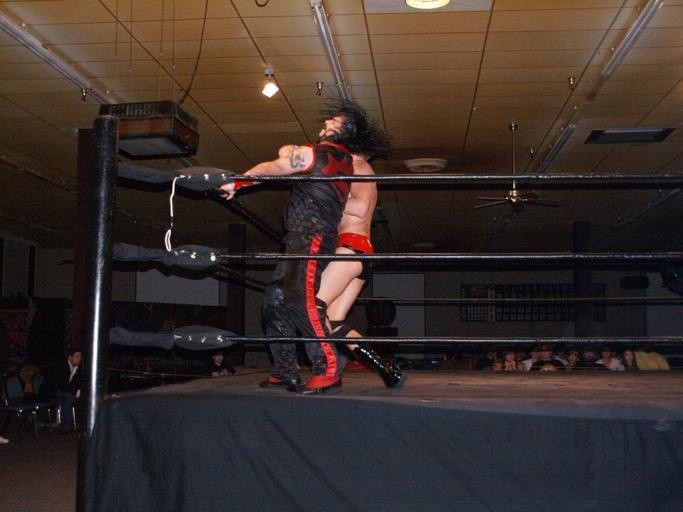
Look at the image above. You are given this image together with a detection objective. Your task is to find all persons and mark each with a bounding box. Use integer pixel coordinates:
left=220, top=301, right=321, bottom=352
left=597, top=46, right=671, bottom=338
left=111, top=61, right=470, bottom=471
left=47, top=347, right=91, bottom=432
left=314, top=131, right=409, bottom=391
left=473, top=346, right=670, bottom=372
left=207, top=350, right=234, bottom=377
left=216, top=97, right=376, bottom=395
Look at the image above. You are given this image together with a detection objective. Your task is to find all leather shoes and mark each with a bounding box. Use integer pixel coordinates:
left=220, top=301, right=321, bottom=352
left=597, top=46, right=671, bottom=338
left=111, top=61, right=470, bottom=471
left=294, top=375, right=342, bottom=396
left=256, top=371, right=302, bottom=391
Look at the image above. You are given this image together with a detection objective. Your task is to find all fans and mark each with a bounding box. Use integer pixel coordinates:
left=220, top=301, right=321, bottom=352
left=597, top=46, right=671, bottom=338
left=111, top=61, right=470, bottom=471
left=473, top=122, right=560, bottom=210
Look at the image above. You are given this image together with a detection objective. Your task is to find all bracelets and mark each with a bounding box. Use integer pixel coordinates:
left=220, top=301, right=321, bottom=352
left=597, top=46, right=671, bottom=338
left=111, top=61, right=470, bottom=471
left=232, top=174, right=254, bottom=193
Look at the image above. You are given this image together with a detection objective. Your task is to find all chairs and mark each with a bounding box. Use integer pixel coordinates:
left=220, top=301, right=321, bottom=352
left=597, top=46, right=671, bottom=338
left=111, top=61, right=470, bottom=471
left=0, top=364, right=76, bottom=442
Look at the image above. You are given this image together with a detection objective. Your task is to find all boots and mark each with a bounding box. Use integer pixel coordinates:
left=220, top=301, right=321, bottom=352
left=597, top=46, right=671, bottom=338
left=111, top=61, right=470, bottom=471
left=350, top=337, right=408, bottom=392
